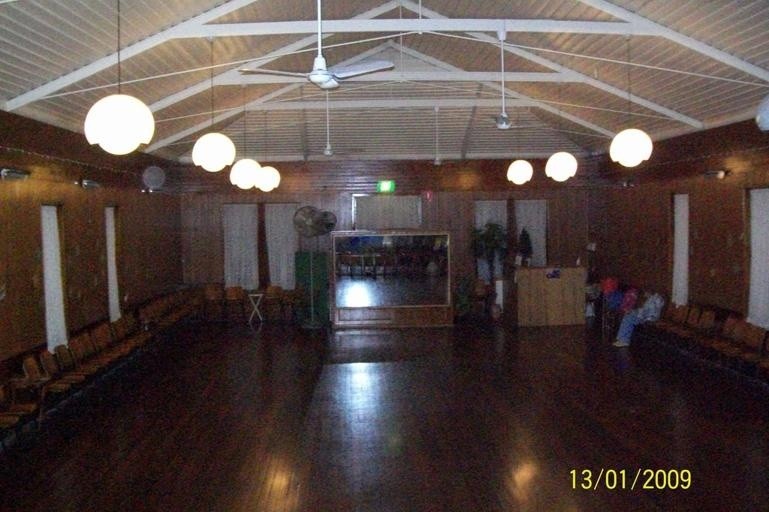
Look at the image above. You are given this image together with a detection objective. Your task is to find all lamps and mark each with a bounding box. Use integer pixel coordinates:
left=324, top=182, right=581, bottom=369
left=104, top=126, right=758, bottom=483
left=192, top=38, right=236, bottom=173
left=506, top=106, right=534, bottom=186
left=609, top=34, right=653, bottom=168
left=83, top=0, right=154, bottom=157
left=228, top=85, right=282, bottom=192
left=544, top=82, right=579, bottom=183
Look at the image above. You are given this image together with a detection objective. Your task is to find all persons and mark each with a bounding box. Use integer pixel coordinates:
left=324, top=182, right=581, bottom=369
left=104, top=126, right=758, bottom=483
left=612, top=285, right=665, bottom=348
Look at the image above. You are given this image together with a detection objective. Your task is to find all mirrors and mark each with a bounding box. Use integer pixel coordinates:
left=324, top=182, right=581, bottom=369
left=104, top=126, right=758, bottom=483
left=334, top=235, right=450, bottom=308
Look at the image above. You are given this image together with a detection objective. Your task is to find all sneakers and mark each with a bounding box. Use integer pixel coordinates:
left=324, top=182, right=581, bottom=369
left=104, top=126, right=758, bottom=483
left=611, top=340, right=630, bottom=348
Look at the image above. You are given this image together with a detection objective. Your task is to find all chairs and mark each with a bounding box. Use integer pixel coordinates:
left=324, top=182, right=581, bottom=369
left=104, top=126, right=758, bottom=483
left=612, top=282, right=769, bottom=404
left=0, top=283, right=203, bottom=452
left=203, top=282, right=306, bottom=325
left=336, top=236, right=448, bottom=281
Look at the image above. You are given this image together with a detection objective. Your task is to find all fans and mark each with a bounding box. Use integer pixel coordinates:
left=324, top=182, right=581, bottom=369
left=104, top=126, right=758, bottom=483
left=292, top=206, right=337, bottom=329
left=459, top=30, right=549, bottom=131
left=237, top=1, right=395, bottom=89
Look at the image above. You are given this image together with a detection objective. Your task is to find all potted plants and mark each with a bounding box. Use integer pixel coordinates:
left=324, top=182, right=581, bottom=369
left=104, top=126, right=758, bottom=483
left=468, top=220, right=509, bottom=325
left=450, top=274, right=488, bottom=328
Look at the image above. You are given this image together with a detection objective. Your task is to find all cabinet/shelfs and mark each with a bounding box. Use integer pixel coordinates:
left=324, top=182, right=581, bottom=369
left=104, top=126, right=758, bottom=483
left=504, top=261, right=586, bottom=328
